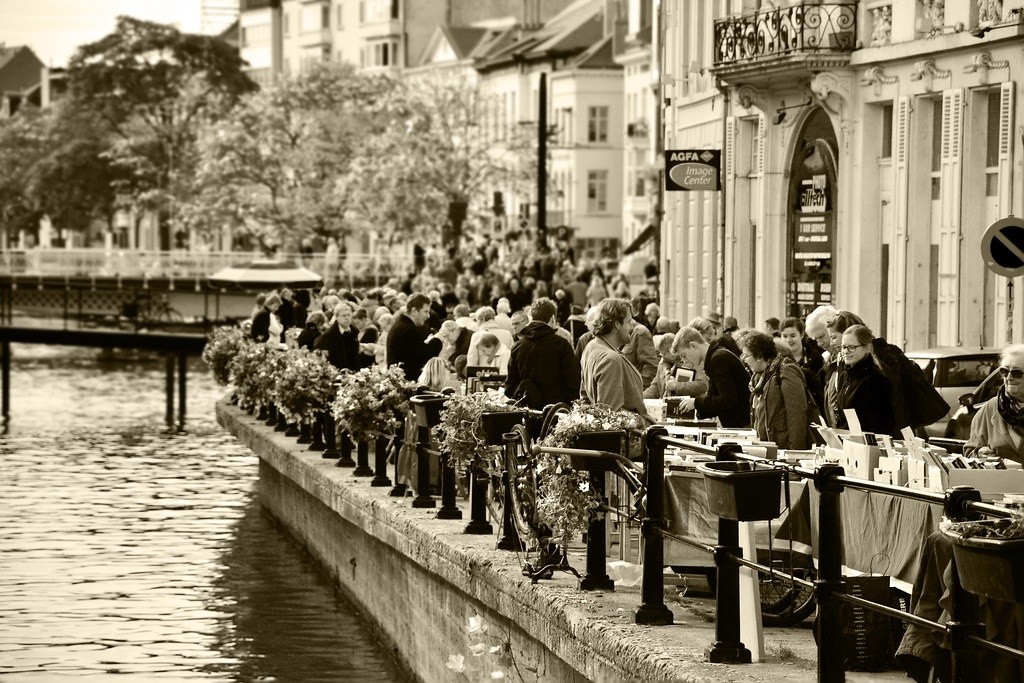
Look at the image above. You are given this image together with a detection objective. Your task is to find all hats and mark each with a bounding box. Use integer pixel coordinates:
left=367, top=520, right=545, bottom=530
left=706, top=312, right=721, bottom=325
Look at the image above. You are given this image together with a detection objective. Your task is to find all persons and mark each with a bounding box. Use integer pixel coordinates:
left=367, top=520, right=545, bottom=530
left=826, top=324, right=894, bottom=445
left=963, top=345, right=1024, bottom=466
left=825, top=308, right=932, bottom=443
left=251, top=210, right=829, bottom=430
left=804, top=305, right=848, bottom=389
left=741, top=332, right=825, bottom=451
left=579, top=297, right=667, bottom=469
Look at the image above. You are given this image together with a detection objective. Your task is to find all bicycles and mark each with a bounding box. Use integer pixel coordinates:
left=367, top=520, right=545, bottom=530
left=81, top=286, right=185, bottom=334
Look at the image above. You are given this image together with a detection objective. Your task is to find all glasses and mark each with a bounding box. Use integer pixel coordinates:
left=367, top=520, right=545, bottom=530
left=999, top=368, right=1024, bottom=379
left=838, top=344, right=863, bottom=353
left=741, top=353, right=752, bottom=359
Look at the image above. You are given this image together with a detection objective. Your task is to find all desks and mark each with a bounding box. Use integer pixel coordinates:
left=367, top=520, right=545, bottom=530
left=603, top=414, right=1024, bottom=683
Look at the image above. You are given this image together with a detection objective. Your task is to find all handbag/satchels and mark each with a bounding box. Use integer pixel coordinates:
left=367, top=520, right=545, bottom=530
left=807, top=400, right=828, bottom=445
